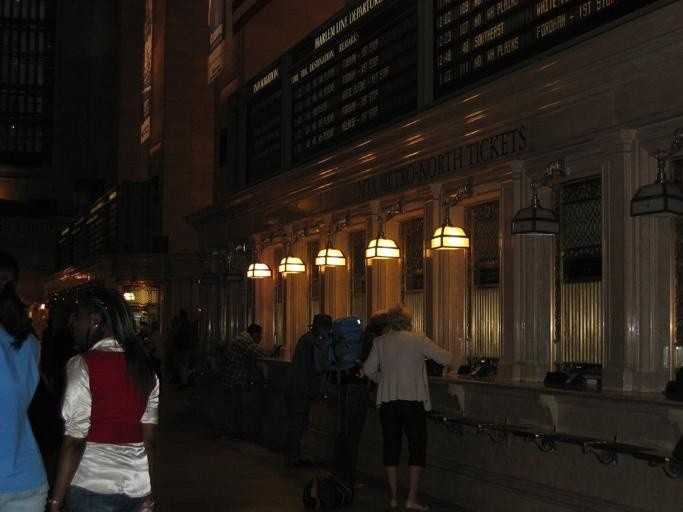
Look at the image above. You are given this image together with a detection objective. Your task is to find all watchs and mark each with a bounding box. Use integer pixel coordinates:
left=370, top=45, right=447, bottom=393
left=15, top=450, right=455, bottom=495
left=49, top=498, right=63, bottom=504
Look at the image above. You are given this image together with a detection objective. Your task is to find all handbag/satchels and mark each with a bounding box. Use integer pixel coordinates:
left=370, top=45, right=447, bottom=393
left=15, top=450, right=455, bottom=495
left=304, top=473, right=348, bottom=511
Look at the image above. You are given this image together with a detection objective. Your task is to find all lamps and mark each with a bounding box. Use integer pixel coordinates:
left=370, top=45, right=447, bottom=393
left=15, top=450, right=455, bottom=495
left=511, top=161, right=568, bottom=236
left=431, top=183, right=471, bottom=250
left=278, top=228, right=306, bottom=272
left=630, top=128, right=683, bottom=217
left=364, top=202, right=401, bottom=259
left=315, top=214, right=346, bottom=266
left=192, top=251, right=219, bottom=285
left=219, top=245, right=243, bottom=282
left=247, top=237, right=272, bottom=278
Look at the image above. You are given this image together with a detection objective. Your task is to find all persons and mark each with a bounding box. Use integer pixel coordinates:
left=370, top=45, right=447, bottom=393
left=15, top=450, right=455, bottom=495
left=325, top=310, right=388, bottom=476
left=0, top=251, right=49, bottom=511
left=222, top=322, right=282, bottom=439
left=281, top=314, right=333, bottom=467
left=42, top=317, right=73, bottom=352
left=359, top=302, right=458, bottom=512
left=50, top=287, right=160, bottom=511
left=139, top=308, right=201, bottom=390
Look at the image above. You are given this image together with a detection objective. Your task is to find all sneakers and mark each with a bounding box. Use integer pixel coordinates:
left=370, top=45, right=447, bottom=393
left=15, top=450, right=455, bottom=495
left=406, top=500, right=429, bottom=510
left=388, top=498, right=396, bottom=507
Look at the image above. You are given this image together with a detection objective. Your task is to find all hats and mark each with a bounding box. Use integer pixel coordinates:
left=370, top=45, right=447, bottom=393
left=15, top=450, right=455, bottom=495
left=307, top=314, right=332, bottom=330
